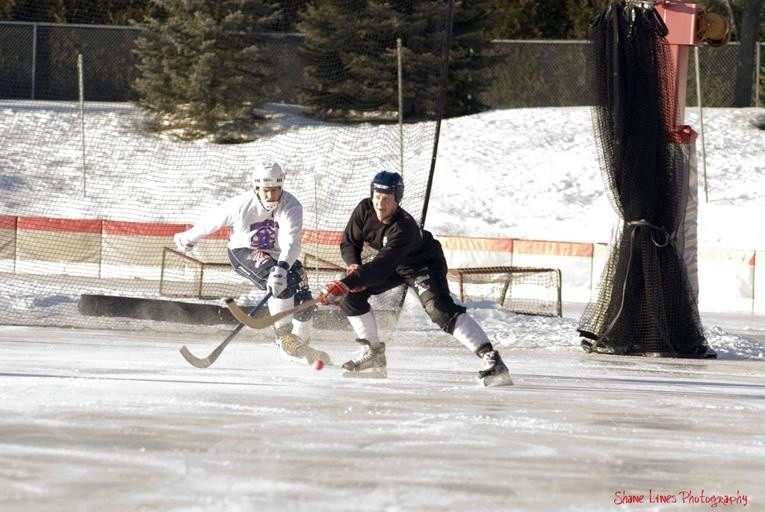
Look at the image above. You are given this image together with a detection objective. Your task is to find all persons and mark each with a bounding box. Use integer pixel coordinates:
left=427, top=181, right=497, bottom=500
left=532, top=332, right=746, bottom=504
left=173, top=160, right=511, bottom=386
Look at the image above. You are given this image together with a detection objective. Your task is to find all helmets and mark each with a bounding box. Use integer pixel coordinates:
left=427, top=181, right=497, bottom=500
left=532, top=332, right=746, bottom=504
left=371, top=171, right=405, bottom=202
left=252, top=160, right=284, bottom=192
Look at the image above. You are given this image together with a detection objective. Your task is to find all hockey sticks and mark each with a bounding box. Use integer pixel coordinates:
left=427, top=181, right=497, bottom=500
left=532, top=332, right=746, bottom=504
left=179, top=291, right=272, bottom=368
left=224, top=297, right=321, bottom=328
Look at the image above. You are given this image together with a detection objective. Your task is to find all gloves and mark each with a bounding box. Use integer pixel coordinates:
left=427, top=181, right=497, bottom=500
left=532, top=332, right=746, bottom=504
left=347, top=264, right=367, bottom=292
left=175, top=232, right=194, bottom=253
left=320, top=280, right=348, bottom=308
left=267, top=266, right=288, bottom=297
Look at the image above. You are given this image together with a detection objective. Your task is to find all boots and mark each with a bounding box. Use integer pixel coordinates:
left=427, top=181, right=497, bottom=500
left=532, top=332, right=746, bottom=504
left=478, top=350, right=509, bottom=380
left=282, top=334, right=330, bottom=364
left=342, top=338, right=386, bottom=372
